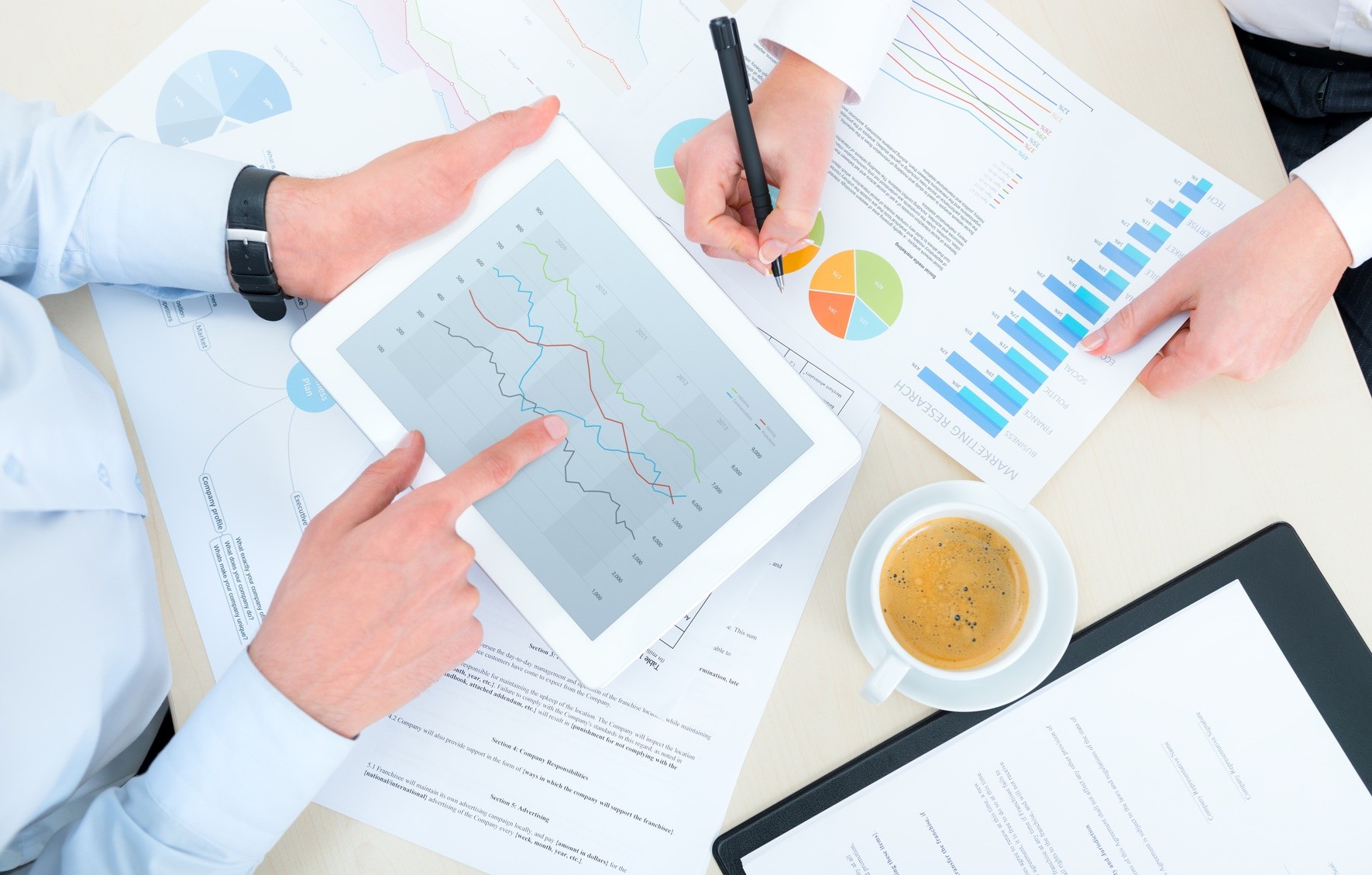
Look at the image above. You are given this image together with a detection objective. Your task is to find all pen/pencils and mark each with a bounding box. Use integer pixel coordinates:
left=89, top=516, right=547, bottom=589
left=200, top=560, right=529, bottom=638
left=709, top=15, right=786, bottom=295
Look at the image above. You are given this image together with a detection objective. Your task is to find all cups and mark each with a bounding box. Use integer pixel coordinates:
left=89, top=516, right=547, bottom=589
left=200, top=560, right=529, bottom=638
left=864, top=504, right=1050, bottom=699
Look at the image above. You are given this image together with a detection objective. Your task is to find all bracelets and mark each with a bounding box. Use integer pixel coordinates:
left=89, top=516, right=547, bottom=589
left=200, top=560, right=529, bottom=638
left=227, top=159, right=293, bottom=320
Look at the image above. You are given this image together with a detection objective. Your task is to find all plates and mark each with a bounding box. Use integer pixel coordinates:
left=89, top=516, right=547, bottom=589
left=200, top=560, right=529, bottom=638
left=845, top=479, right=1080, bottom=712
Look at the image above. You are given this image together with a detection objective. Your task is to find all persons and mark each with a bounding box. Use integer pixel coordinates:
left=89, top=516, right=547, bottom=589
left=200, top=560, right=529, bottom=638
left=0, top=84, right=576, bottom=875
left=674, top=0, right=1372, bottom=406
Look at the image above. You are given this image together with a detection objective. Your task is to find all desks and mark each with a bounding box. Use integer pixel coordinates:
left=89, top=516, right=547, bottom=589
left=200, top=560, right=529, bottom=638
left=0, top=6, right=1372, bottom=875
